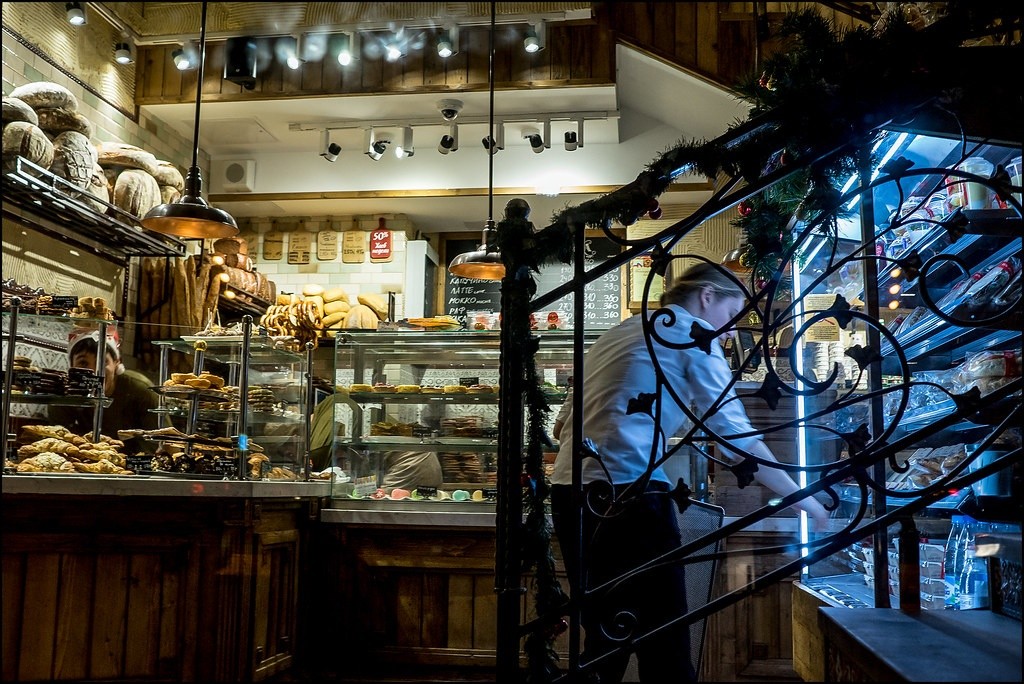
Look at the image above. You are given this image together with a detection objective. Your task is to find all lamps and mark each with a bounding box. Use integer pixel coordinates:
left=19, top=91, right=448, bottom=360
left=438, top=121, right=459, bottom=155
left=338, top=31, right=361, bottom=66
left=448, top=1, right=507, bottom=279
left=526, top=119, right=551, bottom=153
left=320, top=130, right=341, bottom=162
left=287, top=35, right=308, bottom=69
left=364, top=130, right=391, bottom=161
left=65, top=2, right=88, bottom=25
left=523, top=22, right=546, bottom=53
left=396, top=128, right=415, bottom=159
left=172, top=49, right=189, bottom=70
left=565, top=120, right=584, bottom=151
left=437, top=27, right=460, bottom=57
left=141, top=1, right=240, bottom=239
left=482, top=124, right=505, bottom=155
left=115, top=41, right=135, bottom=64
left=385, top=32, right=410, bottom=61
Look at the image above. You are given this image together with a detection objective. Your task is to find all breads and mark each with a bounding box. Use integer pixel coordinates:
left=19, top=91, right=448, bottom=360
left=261, top=282, right=388, bottom=338
left=348, top=382, right=499, bottom=395
left=948, top=348, right=1024, bottom=398
left=2, top=80, right=337, bottom=479
left=906, top=452, right=969, bottom=487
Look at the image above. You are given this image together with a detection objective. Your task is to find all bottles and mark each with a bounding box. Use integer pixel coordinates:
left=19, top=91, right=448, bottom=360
left=943, top=515, right=1021, bottom=610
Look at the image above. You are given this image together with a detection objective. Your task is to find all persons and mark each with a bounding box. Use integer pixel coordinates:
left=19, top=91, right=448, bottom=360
left=263, top=395, right=442, bottom=493
left=49, top=311, right=175, bottom=440
left=550, top=262, right=828, bottom=684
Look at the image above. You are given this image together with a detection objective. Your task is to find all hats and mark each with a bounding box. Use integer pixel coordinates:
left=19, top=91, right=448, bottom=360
left=69, top=323, right=125, bottom=375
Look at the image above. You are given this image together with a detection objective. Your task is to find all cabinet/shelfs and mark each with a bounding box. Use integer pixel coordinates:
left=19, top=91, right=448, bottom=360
left=335, top=330, right=708, bottom=507
left=149, top=337, right=308, bottom=478
left=788, top=129, right=1024, bottom=683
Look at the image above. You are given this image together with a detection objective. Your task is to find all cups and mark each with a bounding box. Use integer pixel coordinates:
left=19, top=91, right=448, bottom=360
left=965, top=443, right=1012, bottom=496
left=1006, top=156, right=1022, bottom=207
left=942, top=157, right=994, bottom=210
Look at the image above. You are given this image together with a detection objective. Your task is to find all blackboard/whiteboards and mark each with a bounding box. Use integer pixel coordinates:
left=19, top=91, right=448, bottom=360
left=437, top=228, right=631, bottom=330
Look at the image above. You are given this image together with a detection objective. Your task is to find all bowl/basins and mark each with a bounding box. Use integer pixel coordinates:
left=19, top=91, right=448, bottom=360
left=879, top=189, right=947, bottom=265
left=815, top=328, right=868, bottom=390
left=827, top=261, right=864, bottom=302
left=669, top=438, right=683, bottom=445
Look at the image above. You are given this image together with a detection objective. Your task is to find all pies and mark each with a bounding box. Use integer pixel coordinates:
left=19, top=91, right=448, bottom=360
left=437, top=415, right=497, bottom=483
left=407, top=317, right=459, bottom=327
